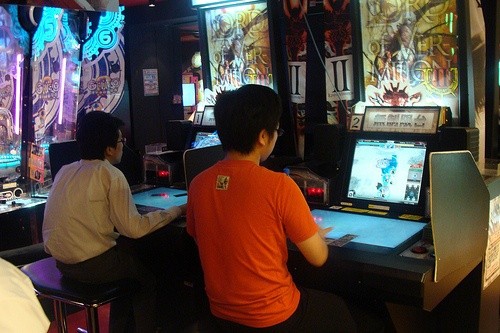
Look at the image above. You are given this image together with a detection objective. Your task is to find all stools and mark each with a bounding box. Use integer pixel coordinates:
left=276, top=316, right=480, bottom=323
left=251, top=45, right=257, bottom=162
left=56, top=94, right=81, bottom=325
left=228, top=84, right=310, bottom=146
left=23, top=257, right=125, bottom=333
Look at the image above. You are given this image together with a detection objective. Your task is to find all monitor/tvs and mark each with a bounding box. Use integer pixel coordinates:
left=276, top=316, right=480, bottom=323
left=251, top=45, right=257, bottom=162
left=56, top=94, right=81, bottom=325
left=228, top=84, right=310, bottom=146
left=343, top=137, right=432, bottom=211
left=187, top=130, right=222, bottom=150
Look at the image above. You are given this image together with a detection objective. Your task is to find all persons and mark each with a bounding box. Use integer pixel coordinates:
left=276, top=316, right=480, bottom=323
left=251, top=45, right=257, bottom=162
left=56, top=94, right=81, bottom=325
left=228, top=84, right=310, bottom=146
left=43, top=110, right=199, bottom=333
left=187, top=84, right=387, bottom=333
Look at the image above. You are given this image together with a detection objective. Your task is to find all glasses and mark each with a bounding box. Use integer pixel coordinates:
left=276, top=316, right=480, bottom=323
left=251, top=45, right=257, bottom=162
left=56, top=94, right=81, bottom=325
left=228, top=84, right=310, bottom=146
left=116, top=138, right=126, bottom=145
left=274, top=128, right=284, bottom=136
